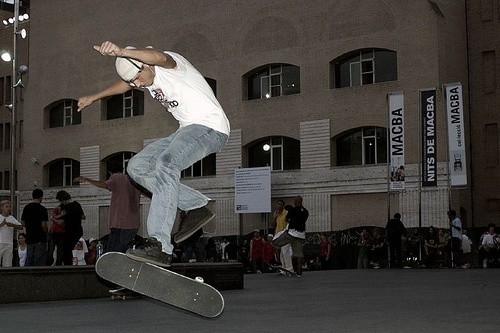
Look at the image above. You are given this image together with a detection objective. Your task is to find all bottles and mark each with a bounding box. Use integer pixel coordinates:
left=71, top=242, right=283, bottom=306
left=96, top=240, right=103, bottom=260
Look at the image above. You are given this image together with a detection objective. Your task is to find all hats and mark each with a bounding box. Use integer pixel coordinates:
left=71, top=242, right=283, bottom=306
left=115, top=46, right=143, bottom=81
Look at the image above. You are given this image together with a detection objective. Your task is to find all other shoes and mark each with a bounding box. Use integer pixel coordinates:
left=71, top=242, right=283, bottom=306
left=483, top=259, right=487, bottom=267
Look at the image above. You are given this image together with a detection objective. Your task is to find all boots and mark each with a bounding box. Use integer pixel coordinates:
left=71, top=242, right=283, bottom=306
left=292, top=255, right=304, bottom=277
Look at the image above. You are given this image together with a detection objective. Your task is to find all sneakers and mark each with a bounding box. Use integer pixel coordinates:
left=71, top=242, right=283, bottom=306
left=174, top=206, right=215, bottom=243
left=126, top=237, right=171, bottom=267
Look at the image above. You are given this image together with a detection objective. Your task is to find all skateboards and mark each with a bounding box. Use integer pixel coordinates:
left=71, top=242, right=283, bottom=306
left=95, top=252, right=225, bottom=318
left=268, top=264, right=304, bottom=278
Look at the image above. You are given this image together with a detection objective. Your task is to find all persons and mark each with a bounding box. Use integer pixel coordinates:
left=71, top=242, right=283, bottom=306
left=369, top=228, right=384, bottom=269
left=273, top=199, right=294, bottom=277
left=447, top=209, right=471, bottom=268
left=400, top=226, right=473, bottom=268
left=0, top=200, right=23, bottom=267
left=218, top=229, right=331, bottom=274
left=272, top=195, right=309, bottom=277
left=479, top=223, right=500, bottom=269
left=21, top=189, right=50, bottom=266
left=71, top=237, right=88, bottom=266
left=49, top=203, right=67, bottom=267
left=1, top=238, right=20, bottom=267
left=51, top=190, right=86, bottom=266
left=18, top=233, right=28, bottom=267
left=358, top=228, right=371, bottom=269
left=77, top=40, right=231, bottom=268
left=84, top=238, right=97, bottom=265
left=73, top=159, right=152, bottom=292
left=385, top=212, right=409, bottom=269
